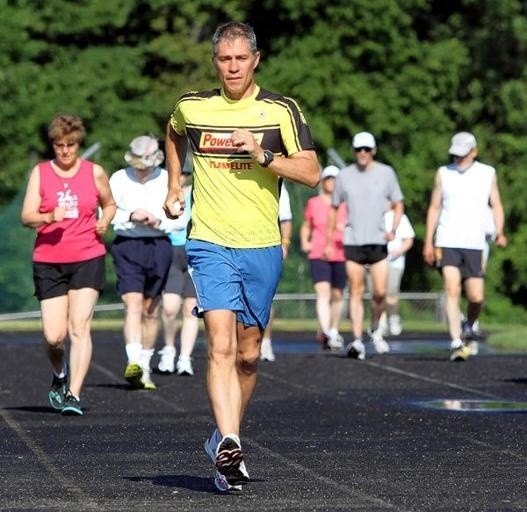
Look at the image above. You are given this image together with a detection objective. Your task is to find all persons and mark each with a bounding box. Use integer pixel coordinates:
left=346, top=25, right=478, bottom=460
left=421, top=130, right=509, bottom=362
left=364, top=195, right=417, bottom=340
left=298, top=164, right=349, bottom=352
left=322, top=131, right=405, bottom=362
left=155, top=158, right=205, bottom=377
left=96, top=134, right=183, bottom=392
left=161, top=18, right=322, bottom=492
left=255, top=178, right=293, bottom=363
left=20, top=110, right=119, bottom=416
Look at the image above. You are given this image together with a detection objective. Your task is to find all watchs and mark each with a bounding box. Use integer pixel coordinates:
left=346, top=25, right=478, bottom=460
left=258, top=149, right=275, bottom=170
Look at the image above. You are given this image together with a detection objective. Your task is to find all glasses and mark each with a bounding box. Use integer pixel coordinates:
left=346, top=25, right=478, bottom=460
left=354, top=147, right=373, bottom=152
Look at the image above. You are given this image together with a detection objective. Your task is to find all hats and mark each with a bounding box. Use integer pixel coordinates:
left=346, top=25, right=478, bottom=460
left=321, top=165, right=340, bottom=180
left=352, top=132, right=376, bottom=149
left=124, top=136, right=164, bottom=170
left=448, top=131, right=476, bottom=157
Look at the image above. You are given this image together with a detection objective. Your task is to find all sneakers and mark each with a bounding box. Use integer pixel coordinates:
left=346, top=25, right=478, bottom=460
left=158, top=346, right=176, bottom=373
left=204, top=429, right=243, bottom=492
left=140, top=378, right=156, bottom=390
left=124, top=363, right=145, bottom=388
left=49, top=360, right=68, bottom=410
left=260, top=338, right=275, bottom=361
left=61, top=395, right=83, bottom=415
left=176, top=356, right=194, bottom=376
left=215, top=436, right=251, bottom=485
left=317, top=313, right=478, bottom=362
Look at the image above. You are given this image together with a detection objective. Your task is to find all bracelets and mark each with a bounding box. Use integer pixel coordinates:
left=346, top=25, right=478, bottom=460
left=281, top=238, right=291, bottom=247
left=391, top=229, right=397, bottom=234
